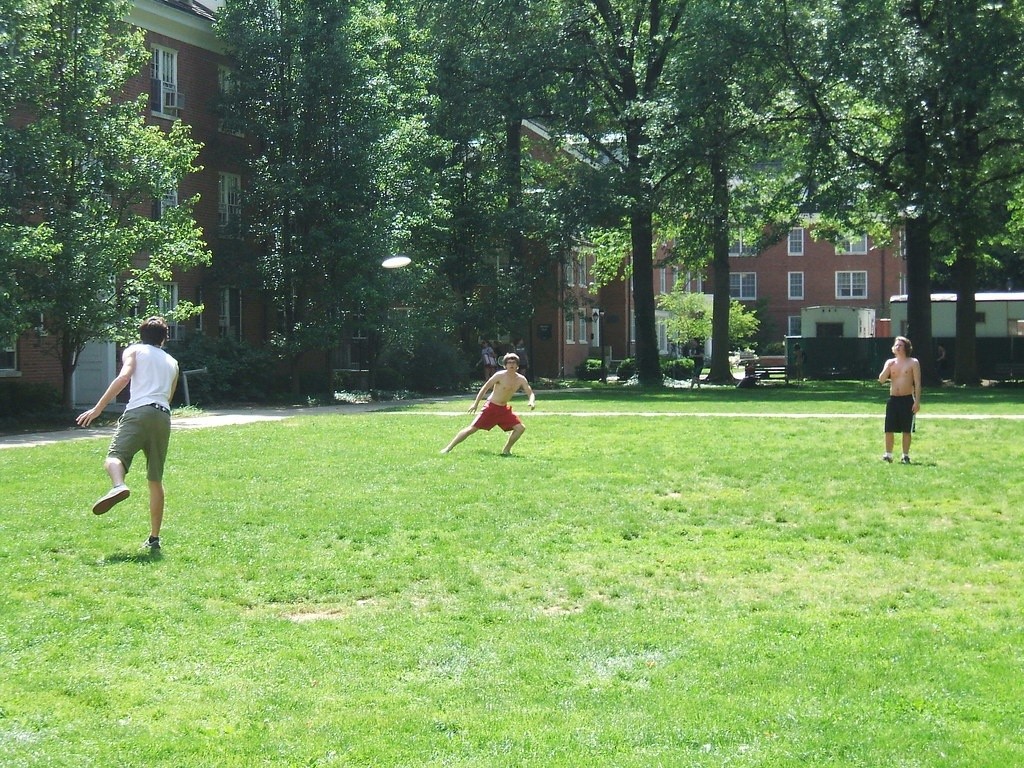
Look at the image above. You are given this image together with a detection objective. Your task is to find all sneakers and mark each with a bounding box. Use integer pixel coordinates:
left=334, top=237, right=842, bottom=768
left=93, top=485, right=130, bottom=516
left=143, top=538, right=162, bottom=549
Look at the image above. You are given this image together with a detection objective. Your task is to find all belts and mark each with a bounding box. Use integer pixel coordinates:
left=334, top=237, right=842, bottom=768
left=151, top=403, right=171, bottom=416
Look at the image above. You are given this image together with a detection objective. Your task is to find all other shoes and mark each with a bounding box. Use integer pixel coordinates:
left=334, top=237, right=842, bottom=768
left=882, top=456, right=893, bottom=463
left=900, top=456, right=911, bottom=465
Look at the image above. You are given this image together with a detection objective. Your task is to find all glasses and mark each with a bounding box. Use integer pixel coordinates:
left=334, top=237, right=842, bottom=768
left=506, top=361, right=518, bottom=364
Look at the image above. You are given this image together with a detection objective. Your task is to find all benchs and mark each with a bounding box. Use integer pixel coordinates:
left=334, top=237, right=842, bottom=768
left=744, top=365, right=788, bottom=386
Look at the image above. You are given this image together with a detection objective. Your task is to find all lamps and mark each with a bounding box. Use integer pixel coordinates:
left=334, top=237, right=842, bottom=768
left=588, top=311, right=598, bottom=323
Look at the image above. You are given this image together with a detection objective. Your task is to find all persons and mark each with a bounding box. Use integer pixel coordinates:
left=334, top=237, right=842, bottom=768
left=878, top=336, right=922, bottom=464
left=793, top=343, right=807, bottom=386
left=688, top=338, right=704, bottom=392
left=440, top=353, right=535, bottom=456
left=475, top=339, right=497, bottom=392
left=511, top=337, right=529, bottom=390
left=76, top=316, right=180, bottom=550
